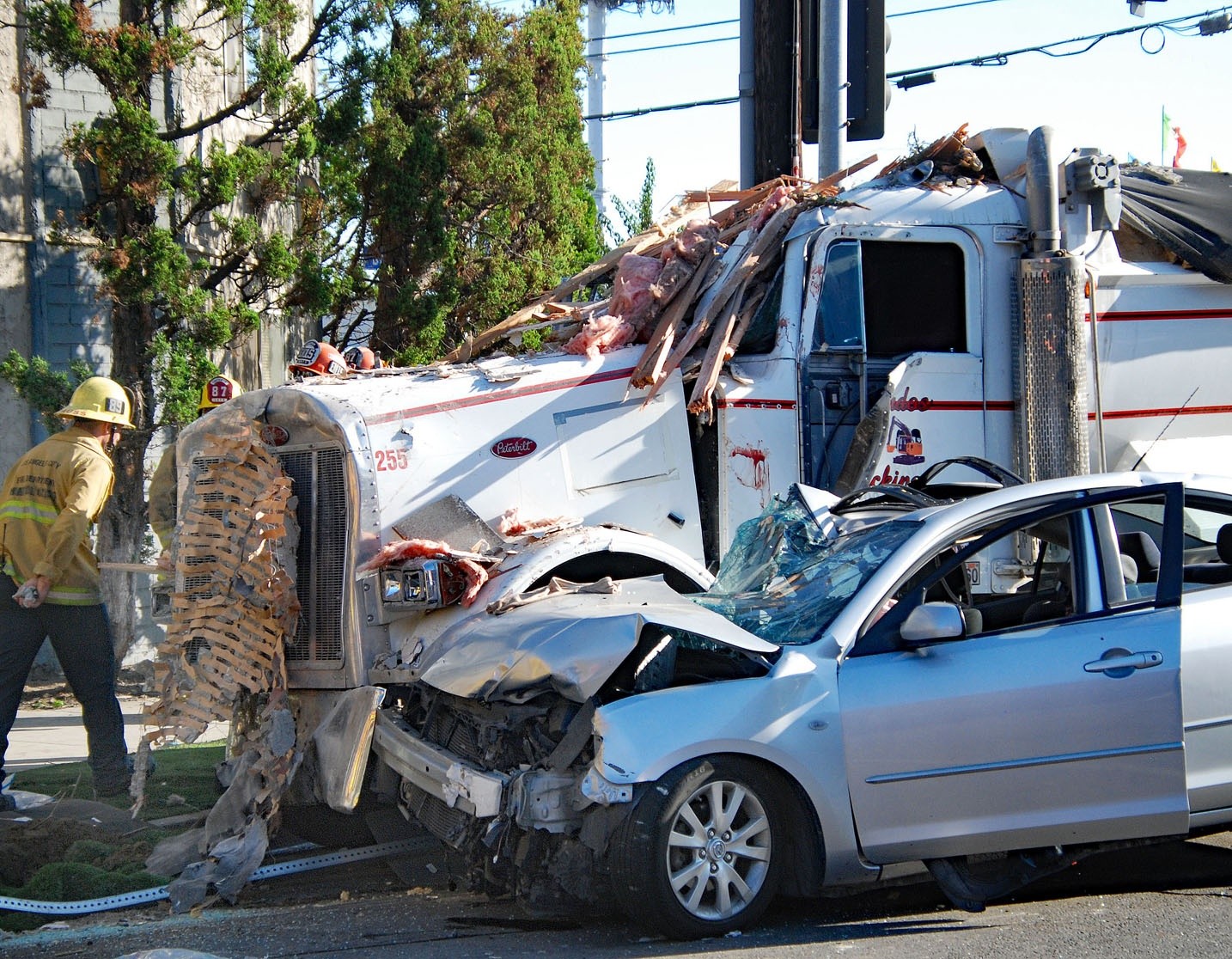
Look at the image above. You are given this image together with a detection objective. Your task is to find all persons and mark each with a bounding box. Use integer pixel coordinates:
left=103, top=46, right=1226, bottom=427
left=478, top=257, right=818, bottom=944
left=149, top=375, right=242, bottom=583
left=288, top=339, right=385, bottom=383
left=0, top=377, right=158, bottom=812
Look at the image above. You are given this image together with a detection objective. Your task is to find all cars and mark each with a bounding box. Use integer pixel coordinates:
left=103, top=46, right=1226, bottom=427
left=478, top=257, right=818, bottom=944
left=372, top=472, right=1232, bottom=941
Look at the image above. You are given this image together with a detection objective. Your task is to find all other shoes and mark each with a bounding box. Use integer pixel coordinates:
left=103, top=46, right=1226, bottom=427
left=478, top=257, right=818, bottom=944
left=95, top=753, right=156, bottom=797
left=0, top=790, right=16, bottom=811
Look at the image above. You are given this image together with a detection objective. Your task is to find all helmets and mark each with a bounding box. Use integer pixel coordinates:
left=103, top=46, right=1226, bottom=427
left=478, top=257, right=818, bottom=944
left=289, top=339, right=347, bottom=376
left=197, top=374, right=244, bottom=411
left=340, top=345, right=375, bottom=374
left=53, top=376, right=136, bottom=429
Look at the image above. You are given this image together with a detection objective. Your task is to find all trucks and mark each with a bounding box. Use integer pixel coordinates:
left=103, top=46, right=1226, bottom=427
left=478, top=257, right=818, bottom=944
left=172, top=125, right=1230, bottom=871
